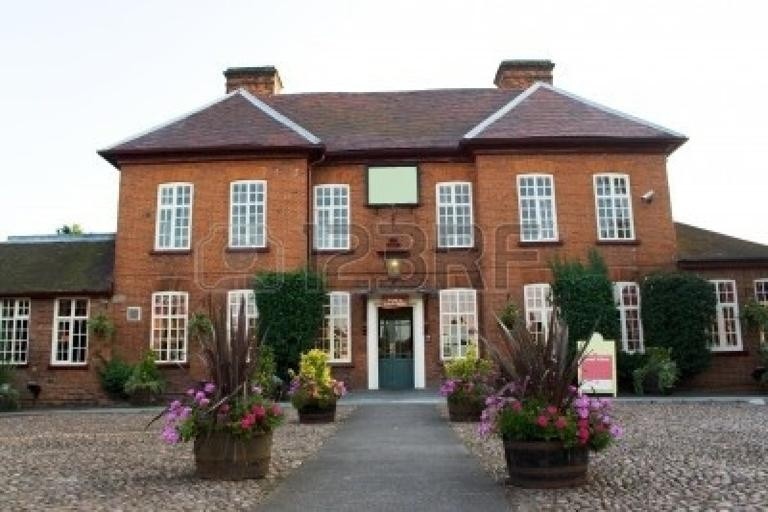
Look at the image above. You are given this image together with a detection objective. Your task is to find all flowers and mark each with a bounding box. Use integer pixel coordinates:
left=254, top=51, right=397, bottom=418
left=440, top=343, right=499, bottom=410
left=474, top=304, right=624, bottom=453
left=145, top=294, right=284, bottom=446
left=287, top=349, right=348, bottom=408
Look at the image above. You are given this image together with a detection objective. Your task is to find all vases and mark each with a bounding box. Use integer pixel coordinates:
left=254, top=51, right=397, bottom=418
left=448, top=399, right=483, bottom=423
left=503, top=439, right=589, bottom=490
left=298, top=405, right=336, bottom=424
left=194, top=434, right=271, bottom=481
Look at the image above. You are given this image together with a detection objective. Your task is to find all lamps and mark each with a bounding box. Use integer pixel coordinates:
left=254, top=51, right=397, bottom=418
left=377, top=250, right=410, bottom=284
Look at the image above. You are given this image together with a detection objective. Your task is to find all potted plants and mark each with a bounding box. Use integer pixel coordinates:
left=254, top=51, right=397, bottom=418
left=0, top=363, right=21, bottom=412
left=632, top=346, right=679, bottom=395
left=124, top=350, right=165, bottom=406
